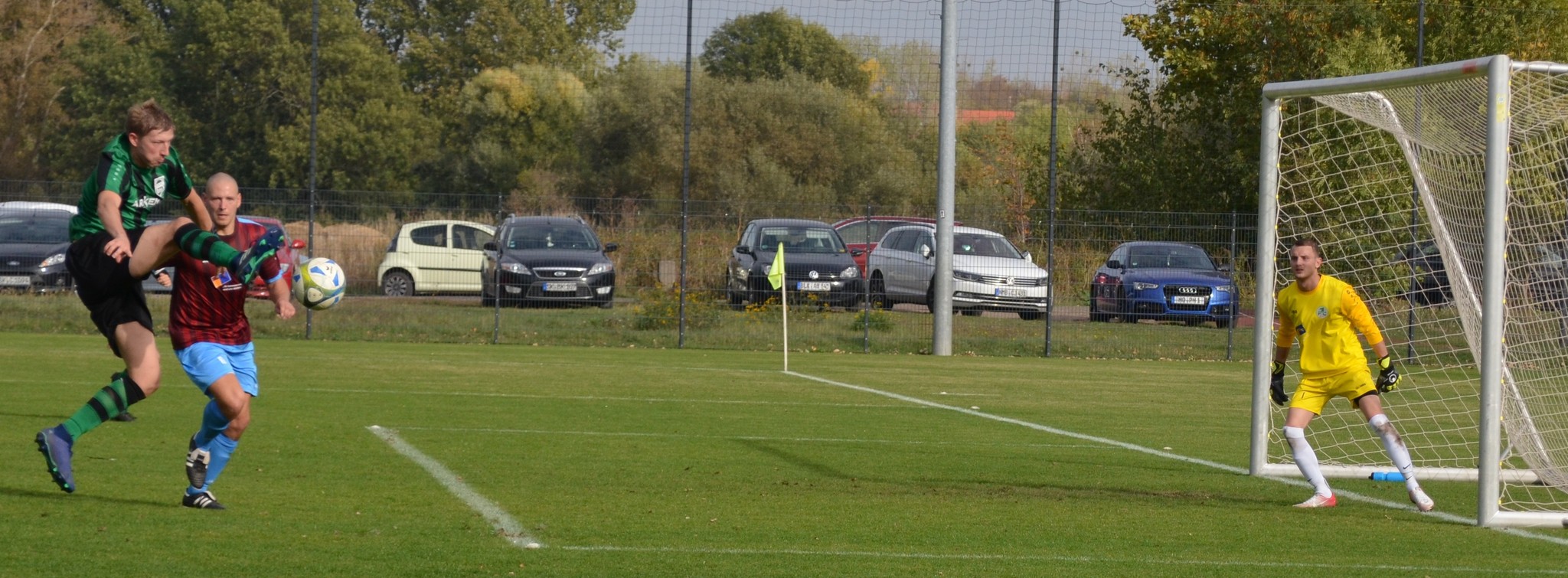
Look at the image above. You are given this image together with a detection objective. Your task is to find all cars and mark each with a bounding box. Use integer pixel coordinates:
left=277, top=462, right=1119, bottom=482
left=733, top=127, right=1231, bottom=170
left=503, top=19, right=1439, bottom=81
left=481, top=213, right=615, bottom=308
left=1089, top=241, right=1249, bottom=327
left=725, top=219, right=864, bottom=311
left=866, top=223, right=1055, bottom=320
left=377, top=220, right=499, bottom=298
left=236, top=214, right=307, bottom=298
left=0, top=208, right=77, bottom=295
left=833, top=218, right=964, bottom=281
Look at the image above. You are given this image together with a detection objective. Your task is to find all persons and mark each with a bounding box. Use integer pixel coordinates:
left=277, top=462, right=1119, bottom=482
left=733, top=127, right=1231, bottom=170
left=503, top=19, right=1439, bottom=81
left=149, top=171, right=296, bottom=510
left=35, top=97, right=285, bottom=494
left=1270, top=239, right=1434, bottom=513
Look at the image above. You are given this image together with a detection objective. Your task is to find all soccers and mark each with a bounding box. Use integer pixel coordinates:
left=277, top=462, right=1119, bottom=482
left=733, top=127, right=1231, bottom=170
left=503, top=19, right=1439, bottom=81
left=293, top=258, right=346, bottom=310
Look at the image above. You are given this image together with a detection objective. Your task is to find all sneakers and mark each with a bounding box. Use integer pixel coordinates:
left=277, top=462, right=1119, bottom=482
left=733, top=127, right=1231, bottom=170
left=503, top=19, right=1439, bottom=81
left=235, top=227, right=286, bottom=286
left=1408, top=487, right=1434, bottom=511
left=1292, top=492, right=1336, bottom=508
left=182, top=489, right=225, bottom=509
left=35, top=427, right=74, bottom=493
left=186, top=430, right=211, bottom=489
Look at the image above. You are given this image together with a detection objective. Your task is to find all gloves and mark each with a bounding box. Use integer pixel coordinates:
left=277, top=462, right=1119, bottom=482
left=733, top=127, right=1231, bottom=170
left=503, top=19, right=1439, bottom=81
left=1375, top=356, right=1402, bottom=394
left=1270, top=361, right=1288, bottom=406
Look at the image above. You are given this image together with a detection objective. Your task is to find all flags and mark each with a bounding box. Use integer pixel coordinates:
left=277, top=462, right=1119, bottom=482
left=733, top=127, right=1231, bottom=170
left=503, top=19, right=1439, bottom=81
left=767, top=242, right=785, bottom=291
